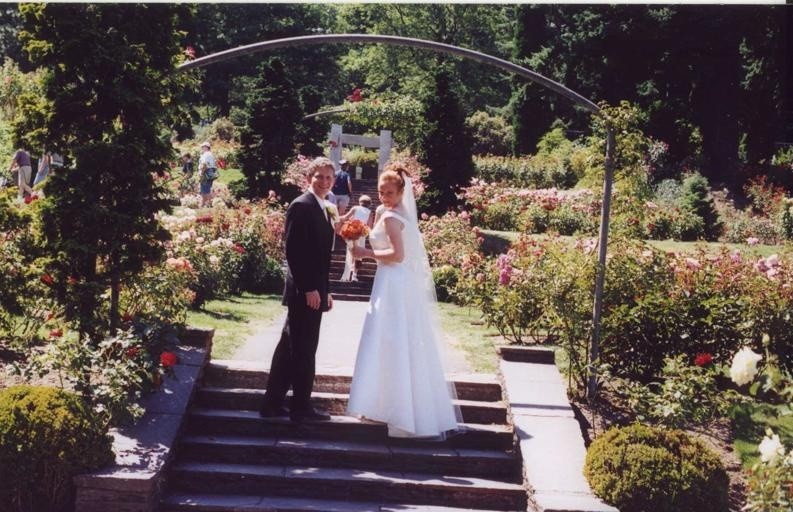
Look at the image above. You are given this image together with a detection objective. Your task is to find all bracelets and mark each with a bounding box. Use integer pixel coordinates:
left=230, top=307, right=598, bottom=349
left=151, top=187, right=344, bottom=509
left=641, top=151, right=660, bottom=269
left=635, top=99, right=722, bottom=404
left=369, top=249, right=374, bottom=257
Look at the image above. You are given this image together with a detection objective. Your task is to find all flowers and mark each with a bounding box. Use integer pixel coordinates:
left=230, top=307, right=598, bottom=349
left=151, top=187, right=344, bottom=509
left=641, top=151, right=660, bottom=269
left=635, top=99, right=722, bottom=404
left=332, top=217, right=371, bottom=280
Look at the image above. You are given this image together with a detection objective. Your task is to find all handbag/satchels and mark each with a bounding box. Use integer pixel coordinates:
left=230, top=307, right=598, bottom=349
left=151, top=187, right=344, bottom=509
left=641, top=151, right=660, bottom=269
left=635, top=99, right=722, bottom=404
left=205, top=168, right=219, bottom=180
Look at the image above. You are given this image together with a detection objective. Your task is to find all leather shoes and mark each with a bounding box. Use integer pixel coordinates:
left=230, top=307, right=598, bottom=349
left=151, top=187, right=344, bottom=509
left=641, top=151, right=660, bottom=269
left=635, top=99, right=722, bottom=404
left=260, top=408, right=290, bottom=417
left=290, top=410, right=330, bottom=421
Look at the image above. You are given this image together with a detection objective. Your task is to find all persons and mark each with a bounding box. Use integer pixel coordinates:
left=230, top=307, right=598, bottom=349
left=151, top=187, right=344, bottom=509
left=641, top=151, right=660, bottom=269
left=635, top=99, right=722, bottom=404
left=324, top=159, right=373, bottom=283
left=260, top=156, right=336, bottom=424
left=344, top=164, right=468, bottom=443
left=181, top=151, right=193, bottom=177
left=197, top=142, right=217, bottom=208
left=7, top=147, right=63, bottom=204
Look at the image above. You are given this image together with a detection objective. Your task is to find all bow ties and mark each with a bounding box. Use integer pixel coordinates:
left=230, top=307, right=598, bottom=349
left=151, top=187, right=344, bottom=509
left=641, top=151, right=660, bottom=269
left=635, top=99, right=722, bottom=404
left=318, top=199, right=336, bottom=210
